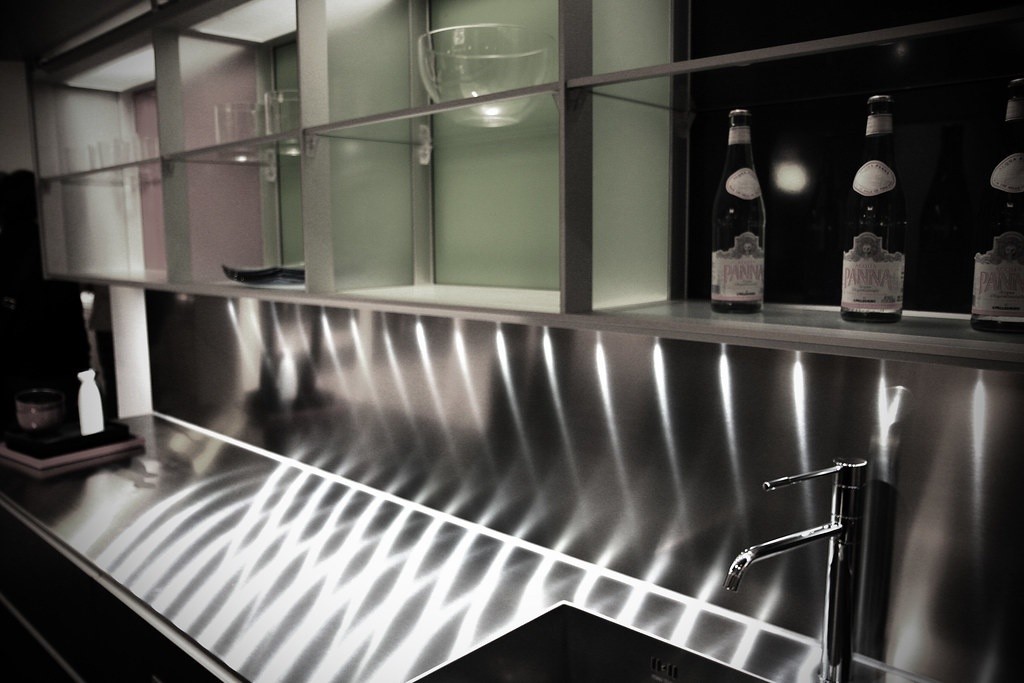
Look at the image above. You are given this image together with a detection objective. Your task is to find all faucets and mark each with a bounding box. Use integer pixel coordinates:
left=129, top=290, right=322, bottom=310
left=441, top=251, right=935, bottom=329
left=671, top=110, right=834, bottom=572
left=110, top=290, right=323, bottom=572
left=724, top=455, right=870, bottom=683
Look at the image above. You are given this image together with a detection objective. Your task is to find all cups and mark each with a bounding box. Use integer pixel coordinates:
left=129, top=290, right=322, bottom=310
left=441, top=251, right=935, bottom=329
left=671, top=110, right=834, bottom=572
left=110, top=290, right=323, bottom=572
left=266, top=92, right=301, bottom=154
left=44, top=134, right=157, bottom=175
left=214, top=102, right=258, bottom=160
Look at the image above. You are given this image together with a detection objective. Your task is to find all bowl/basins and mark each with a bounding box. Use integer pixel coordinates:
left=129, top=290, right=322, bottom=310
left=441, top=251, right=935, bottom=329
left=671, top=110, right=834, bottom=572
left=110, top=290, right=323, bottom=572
left=16, top=389, right=67, bottom=430
left=417, top=26, right=555, bottom=128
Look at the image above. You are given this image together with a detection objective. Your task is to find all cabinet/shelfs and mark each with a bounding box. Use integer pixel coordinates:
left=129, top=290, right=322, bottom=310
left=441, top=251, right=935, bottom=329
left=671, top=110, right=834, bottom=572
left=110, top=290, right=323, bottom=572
left=0, top=0, right=1023, bottom=367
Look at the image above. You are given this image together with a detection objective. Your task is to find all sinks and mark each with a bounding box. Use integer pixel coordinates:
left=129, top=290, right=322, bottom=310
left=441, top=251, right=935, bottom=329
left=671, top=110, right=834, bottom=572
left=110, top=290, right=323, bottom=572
left=402, top=600, right=774, bottom=683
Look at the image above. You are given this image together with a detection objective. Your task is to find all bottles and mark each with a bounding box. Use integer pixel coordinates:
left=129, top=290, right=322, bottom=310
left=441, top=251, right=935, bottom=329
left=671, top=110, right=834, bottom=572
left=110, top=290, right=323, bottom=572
left=970, top=76, right=1024, bottom=333
left=711, top=109, right=765, bottom=314
left=78, top=372, right=104, bottom=435
left=839, top=95, right=904, bottom=322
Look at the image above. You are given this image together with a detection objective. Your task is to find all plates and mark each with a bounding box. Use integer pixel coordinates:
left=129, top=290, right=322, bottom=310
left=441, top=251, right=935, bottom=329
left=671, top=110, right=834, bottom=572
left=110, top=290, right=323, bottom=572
left=223, top=265, right=305, bottom=285
